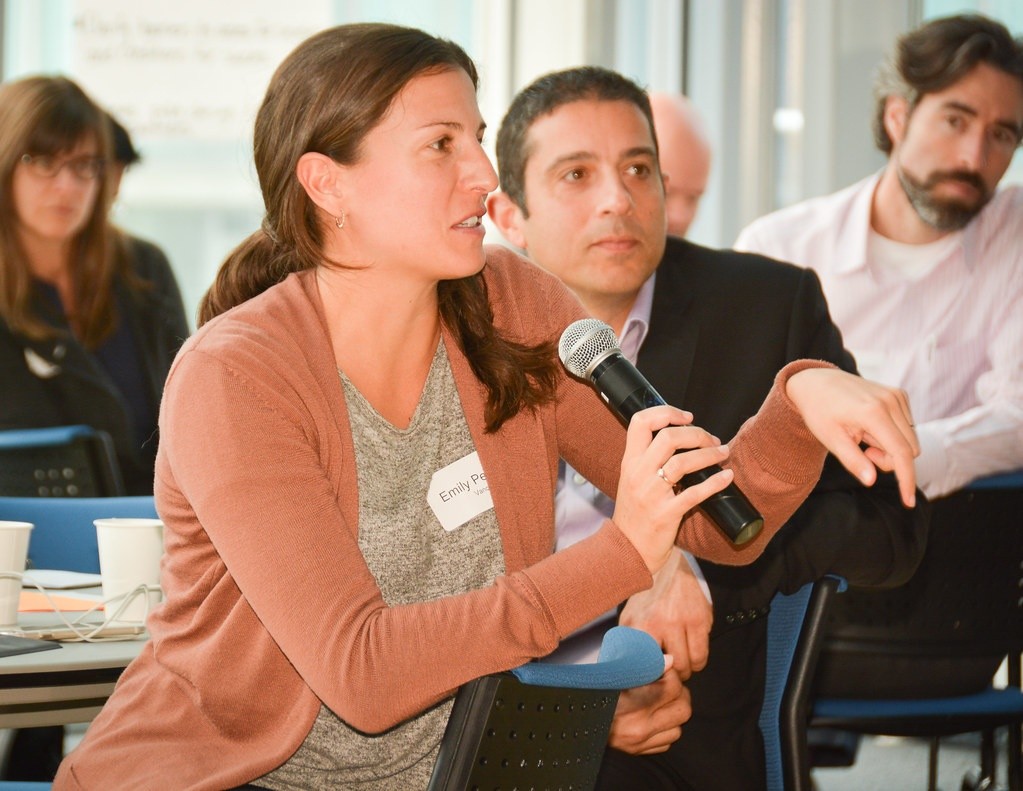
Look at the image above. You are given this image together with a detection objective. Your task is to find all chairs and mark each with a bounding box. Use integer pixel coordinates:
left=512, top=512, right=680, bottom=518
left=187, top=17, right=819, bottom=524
left=1, top=423, right=1023, bottom=789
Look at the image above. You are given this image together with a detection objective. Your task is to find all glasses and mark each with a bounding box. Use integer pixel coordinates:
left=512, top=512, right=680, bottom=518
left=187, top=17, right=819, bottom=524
left=19, top=151, right=103, bottom=180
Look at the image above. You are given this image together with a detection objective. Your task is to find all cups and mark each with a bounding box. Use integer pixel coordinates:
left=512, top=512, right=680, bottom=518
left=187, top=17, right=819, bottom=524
left=94, top=516, right=168, bottom=628
left=0, top=519, right=35, bottom=627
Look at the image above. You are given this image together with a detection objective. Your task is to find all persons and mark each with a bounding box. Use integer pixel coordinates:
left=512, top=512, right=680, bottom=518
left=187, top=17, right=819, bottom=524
left=0, top=74, right=194, bottom=497
left=50, top=12, right=1022, bottom=791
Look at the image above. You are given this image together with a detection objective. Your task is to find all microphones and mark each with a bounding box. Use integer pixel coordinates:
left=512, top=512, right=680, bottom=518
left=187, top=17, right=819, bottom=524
left=557, top=318, right=766, bottom=552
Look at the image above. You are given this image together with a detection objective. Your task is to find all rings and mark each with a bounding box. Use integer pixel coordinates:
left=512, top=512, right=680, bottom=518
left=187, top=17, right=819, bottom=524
left=657, top=467, right=677, bottom=487
